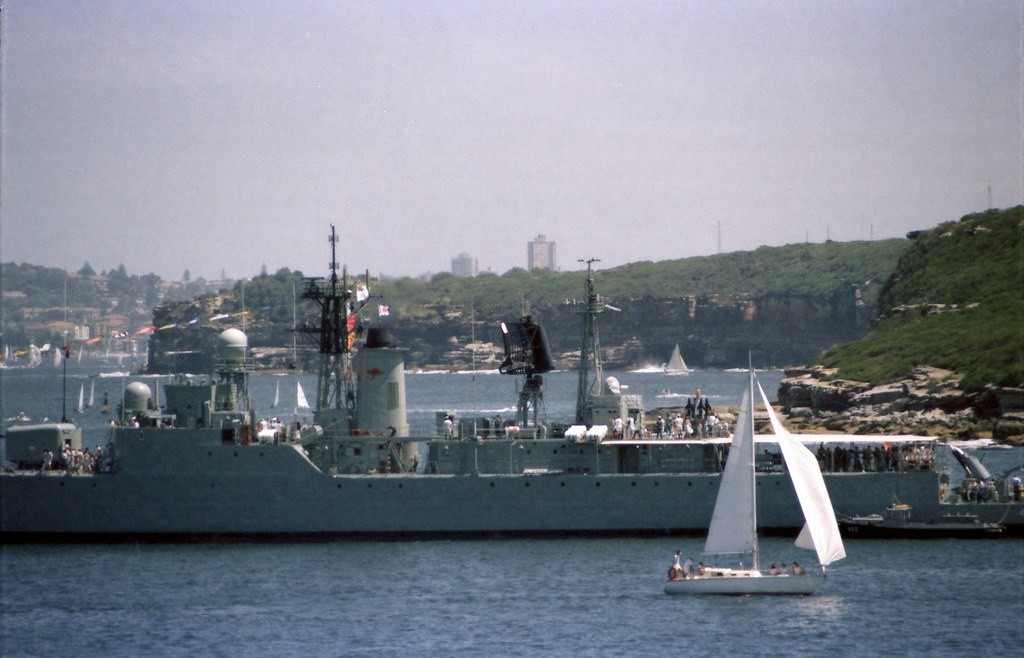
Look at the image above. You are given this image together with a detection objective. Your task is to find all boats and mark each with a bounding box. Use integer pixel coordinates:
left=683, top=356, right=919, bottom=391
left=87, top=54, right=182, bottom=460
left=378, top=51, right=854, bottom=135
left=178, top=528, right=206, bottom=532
left=0, top=224, right=1024, bottom=542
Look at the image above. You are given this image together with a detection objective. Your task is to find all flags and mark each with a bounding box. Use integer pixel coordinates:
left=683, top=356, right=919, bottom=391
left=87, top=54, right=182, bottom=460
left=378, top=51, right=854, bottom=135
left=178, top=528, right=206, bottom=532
left=355, top=284, right=369, bottom=301
left=378, top=304, right=390, bottom=317
left=346, top=302, right=356, bottom=350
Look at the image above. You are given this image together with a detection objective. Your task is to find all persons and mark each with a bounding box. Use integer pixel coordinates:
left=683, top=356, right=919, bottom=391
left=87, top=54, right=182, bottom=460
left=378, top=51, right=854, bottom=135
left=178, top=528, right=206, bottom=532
left=964, top=474, right=1024, bottom=503
left=612, top=412, right=723, bottom=441
left=442, top=415, right=453, bottom=441
left=40, top=444, right=106, bottom=474
left=494, top=414, right=505, bottom=440
left=110, top=416, right=173, bottom=429
left=816, top=442, right=936, bottom=473
left=670, top=550, right=807, bottom=577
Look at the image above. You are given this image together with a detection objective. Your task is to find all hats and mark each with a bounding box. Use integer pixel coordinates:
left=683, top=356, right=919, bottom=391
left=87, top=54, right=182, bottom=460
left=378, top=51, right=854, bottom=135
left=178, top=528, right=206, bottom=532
left=677, top=414, right=681, bottom=416
left=658, top=416, right=662, bottom=418
left=445, top=416, right=449, bottom=418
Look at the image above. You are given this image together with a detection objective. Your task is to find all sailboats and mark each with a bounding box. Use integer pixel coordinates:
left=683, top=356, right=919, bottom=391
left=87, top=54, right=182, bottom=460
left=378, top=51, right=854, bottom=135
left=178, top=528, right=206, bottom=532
left=662, top=349, right=848, bottom=596
left=661, top=344, right=695, bottom=377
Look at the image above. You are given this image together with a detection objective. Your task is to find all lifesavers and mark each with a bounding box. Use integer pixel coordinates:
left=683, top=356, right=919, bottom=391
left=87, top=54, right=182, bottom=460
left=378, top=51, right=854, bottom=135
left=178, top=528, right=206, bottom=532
left=667, top=566, right=678, bottom=580
